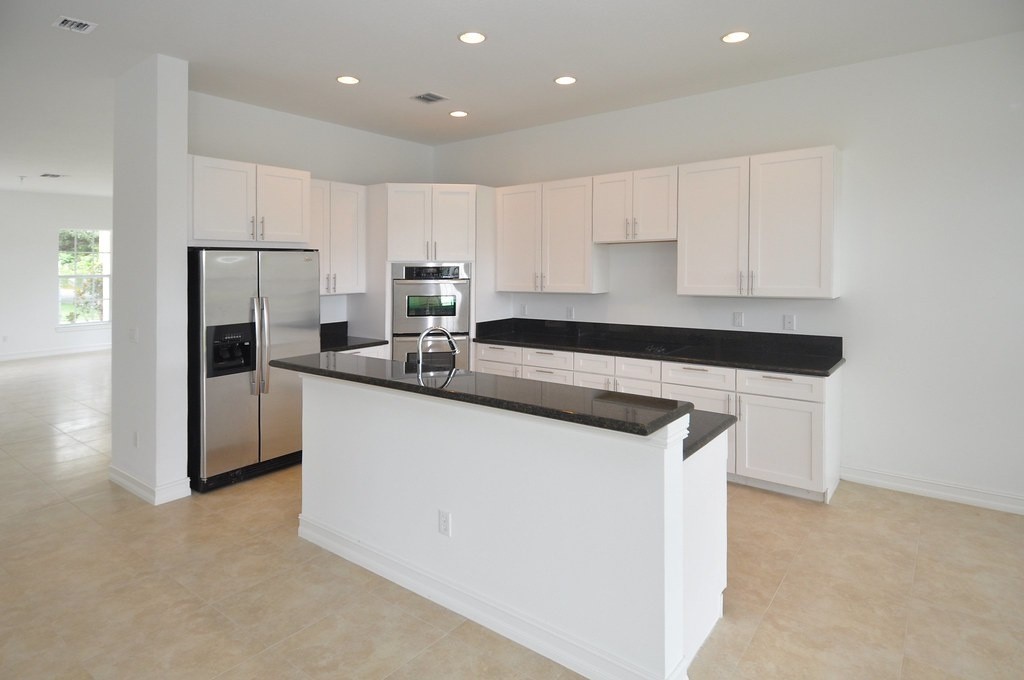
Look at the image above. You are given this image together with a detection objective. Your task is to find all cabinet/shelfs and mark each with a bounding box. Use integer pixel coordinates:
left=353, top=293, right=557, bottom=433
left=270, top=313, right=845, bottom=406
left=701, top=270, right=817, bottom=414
left=475, top=342, right=846, bottom=504
left=334, top=342, right=391, bottom=358
left=186, top=143, right=851, bottom=298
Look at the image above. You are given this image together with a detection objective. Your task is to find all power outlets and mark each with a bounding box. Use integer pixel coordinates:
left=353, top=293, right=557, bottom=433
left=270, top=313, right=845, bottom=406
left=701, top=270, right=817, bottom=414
left=783, top=313, right=795, bottom=331
left=732, top=310, right=744, bottom=328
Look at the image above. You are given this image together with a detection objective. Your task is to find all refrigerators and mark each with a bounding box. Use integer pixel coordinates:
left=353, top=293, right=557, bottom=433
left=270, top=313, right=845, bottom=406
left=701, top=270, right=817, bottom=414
left=187, top=249, right=321, bottom=494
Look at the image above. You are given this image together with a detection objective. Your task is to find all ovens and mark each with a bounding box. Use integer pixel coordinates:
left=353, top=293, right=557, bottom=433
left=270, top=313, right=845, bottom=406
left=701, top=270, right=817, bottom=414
left=391, top=261, right=470, bottom=372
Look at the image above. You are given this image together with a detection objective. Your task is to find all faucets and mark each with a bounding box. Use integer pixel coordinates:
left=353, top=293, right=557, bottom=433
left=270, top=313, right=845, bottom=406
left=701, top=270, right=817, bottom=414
left=417, top=364, right=459, bottom=391
left=416, top=326, right=460, bottom=364
